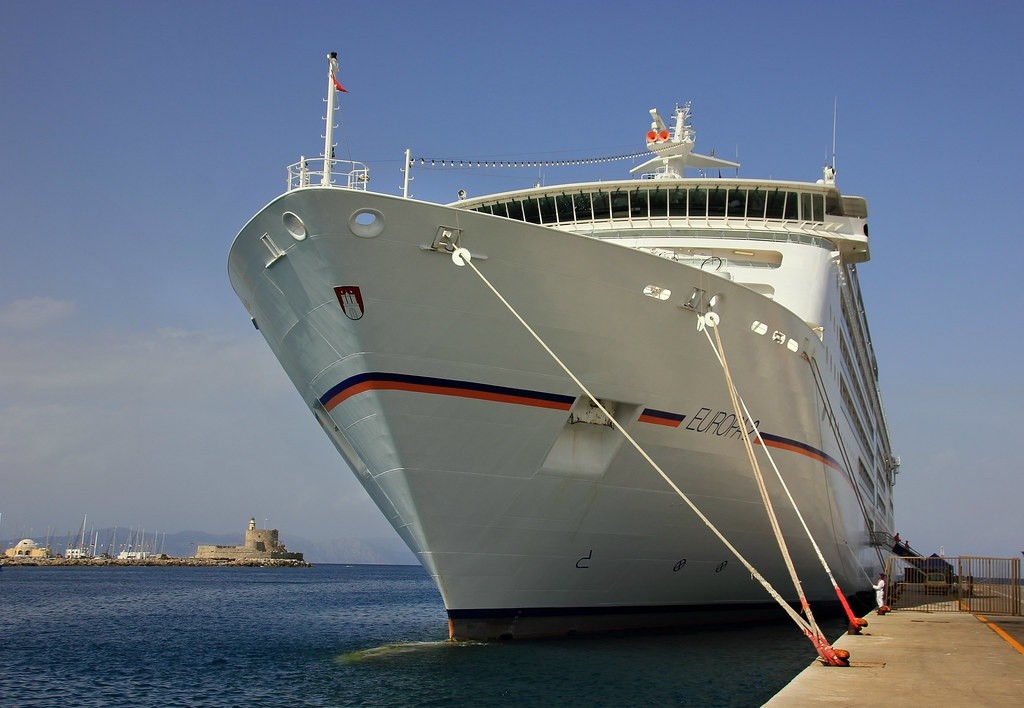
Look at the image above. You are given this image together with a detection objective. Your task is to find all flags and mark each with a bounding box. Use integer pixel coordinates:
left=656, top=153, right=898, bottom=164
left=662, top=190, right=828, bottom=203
left=332, top=73, right=349, bottom=92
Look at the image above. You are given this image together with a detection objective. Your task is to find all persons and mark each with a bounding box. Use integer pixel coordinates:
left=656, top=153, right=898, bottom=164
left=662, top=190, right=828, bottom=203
left=904, top=541, right=910, bottom=549
left=894, top=532, right=900, bottom=542
left=871, top=572, right=885, bottom=611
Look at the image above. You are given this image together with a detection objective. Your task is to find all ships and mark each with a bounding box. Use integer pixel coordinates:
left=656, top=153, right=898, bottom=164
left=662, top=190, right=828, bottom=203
left=225, top=52, right=973, bottom=640
